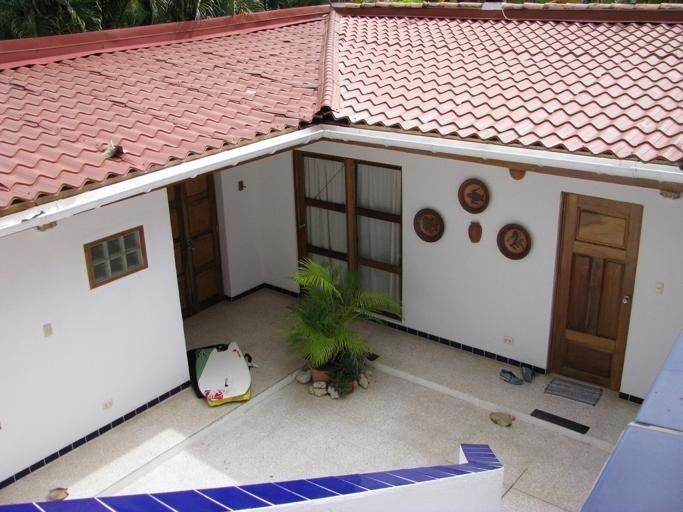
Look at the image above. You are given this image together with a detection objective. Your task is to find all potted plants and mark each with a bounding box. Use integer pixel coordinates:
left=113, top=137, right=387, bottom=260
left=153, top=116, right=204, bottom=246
left=327, top=347, right=366, bottom=396
left=277, top=251, right=404, bottom=384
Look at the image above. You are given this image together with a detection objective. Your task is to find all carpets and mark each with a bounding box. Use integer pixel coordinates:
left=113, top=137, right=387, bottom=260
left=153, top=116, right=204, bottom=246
left=541, top=377, right=604, bottom=408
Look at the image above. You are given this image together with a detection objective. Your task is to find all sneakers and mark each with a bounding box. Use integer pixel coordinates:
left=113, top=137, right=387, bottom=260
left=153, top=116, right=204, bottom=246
left=500, top=369, right=523, bottom=385
left=522, top=367, right=535, bottom=383
